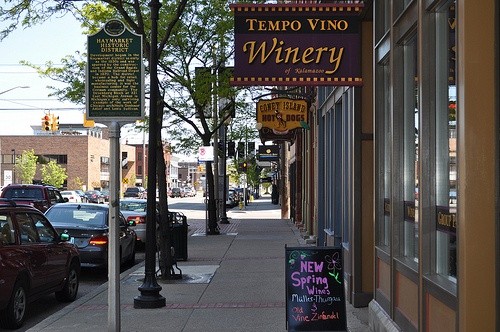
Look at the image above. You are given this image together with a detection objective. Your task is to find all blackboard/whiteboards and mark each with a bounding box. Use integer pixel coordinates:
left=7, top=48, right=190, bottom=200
left=285, top=247, right=347, bottom=332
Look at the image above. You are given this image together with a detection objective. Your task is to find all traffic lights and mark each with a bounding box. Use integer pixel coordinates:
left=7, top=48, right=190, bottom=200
left=53, top=115, right=60, bottom=132
left=41, top=116, right=46, bottom=132
left=44, top=113, right=51, bottom=131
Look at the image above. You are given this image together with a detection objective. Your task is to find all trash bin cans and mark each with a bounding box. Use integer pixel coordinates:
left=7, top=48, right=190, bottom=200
left=155, top=211, right=190, bottom=261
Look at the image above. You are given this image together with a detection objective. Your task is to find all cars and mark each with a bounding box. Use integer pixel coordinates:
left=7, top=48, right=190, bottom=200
left=0, top=203, right=82, bottom=330
left=55, top=187, right=111, bottom=204
left=112, top=197, right=171, bottom=249
left=123, top=186, right=198, bottom=199
left=0, top=182, right=69, bottom=228
left=28, top=202, right=137, bottom=273
left=225, top=185, right=254, bottom=208
